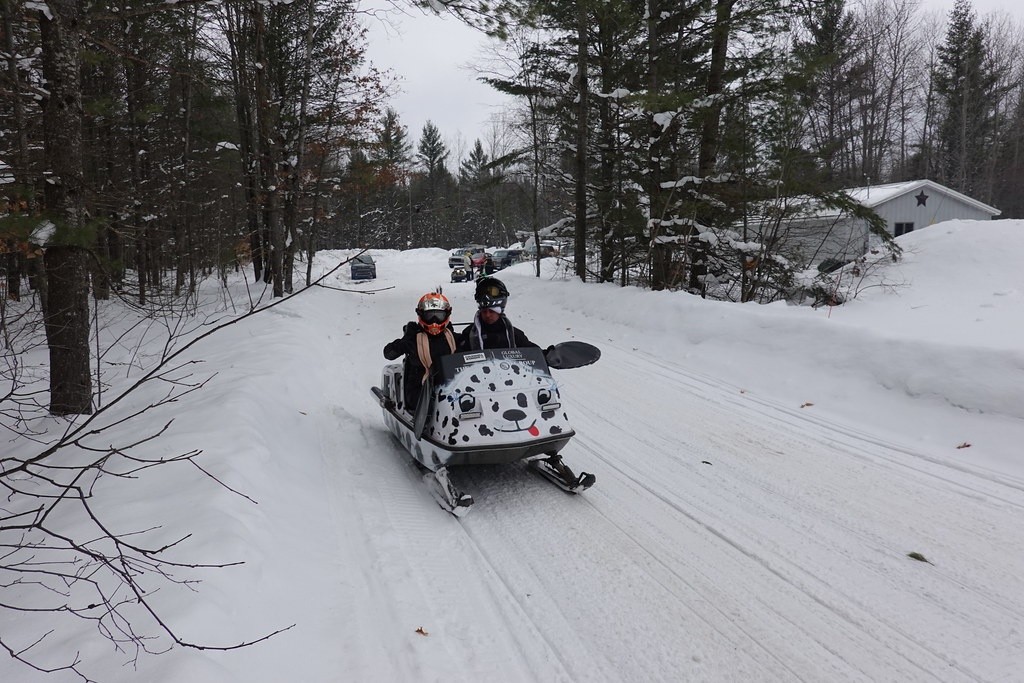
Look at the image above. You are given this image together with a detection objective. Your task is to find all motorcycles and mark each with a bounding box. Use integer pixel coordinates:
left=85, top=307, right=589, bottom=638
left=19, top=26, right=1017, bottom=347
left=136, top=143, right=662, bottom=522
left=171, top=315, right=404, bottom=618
left=366, top=319, right=602, bottom=516
left=450, top=267, right=473, bottom=283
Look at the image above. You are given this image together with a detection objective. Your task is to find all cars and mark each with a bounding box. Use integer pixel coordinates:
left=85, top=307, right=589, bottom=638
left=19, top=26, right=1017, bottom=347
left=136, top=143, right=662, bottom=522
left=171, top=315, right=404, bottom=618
left=472, top=252, right=492, bottom=266
left=531, top=244, right=559, bottom=259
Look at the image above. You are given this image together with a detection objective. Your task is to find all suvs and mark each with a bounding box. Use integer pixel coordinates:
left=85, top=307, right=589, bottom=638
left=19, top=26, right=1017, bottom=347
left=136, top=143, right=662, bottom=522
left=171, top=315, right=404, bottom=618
left=492, top=249, right=531, bottom=269
left=350, top=255, right=377, bottom=280
left=447, top=248, right=486, bottom=268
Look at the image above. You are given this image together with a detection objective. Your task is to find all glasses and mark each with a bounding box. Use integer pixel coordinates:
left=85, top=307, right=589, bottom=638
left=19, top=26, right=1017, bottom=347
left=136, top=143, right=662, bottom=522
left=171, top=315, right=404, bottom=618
left=416, top=308, right=448, bottom=325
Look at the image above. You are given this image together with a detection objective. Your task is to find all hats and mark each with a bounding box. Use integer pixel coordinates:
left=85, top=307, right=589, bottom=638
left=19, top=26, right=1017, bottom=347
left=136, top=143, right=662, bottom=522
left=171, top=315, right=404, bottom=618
left=488, top=307, right=501, bottom=314
left=468, top=253, right=471, bottom=257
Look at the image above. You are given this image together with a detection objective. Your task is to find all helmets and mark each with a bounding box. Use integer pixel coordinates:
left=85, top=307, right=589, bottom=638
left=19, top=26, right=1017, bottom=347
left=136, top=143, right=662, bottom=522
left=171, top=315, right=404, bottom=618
left=474, top=277, right=510, bottom=314
left=416, top=293, right=452, bottom=335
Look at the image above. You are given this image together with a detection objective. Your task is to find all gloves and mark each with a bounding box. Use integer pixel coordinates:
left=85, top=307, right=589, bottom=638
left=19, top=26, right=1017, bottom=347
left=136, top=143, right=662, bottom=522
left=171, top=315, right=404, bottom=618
left=544, top=345, right=557, bottom=368
left=397, top=339, right=413, bottom=353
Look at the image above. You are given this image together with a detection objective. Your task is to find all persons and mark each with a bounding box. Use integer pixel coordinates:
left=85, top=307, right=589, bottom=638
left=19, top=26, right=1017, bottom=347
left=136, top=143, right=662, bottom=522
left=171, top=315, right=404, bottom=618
left=384, top=292, right=467, bottom=414
left=461, top=277, right=556, bottom=357
left=464, top=253, right=474, bottom=281
left=485, top=259, right=498, bottom=275
